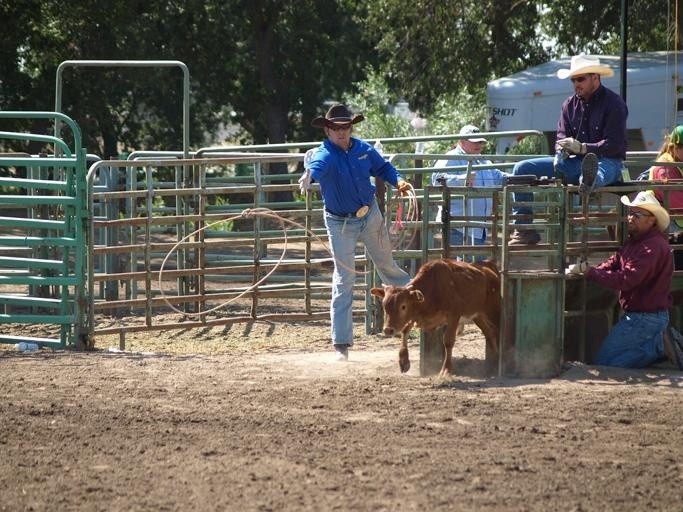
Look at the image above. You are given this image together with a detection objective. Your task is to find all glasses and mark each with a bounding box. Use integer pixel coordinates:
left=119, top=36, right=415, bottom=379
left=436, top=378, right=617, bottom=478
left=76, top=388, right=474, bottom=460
left=570, top=76, right=584, bottom=84
left=327, top=123, right=349, bottom=132
left=628, top=209, right=654, bottom=220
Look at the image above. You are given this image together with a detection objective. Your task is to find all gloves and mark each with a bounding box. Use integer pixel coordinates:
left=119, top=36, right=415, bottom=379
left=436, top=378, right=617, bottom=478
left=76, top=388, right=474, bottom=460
left=397, top=182, right=408, bottom=192
left=299, top=168, right=313, bottom=195
left=556, top=136, right=587, bottom=155
left=570, top=261, right=587, bottom=274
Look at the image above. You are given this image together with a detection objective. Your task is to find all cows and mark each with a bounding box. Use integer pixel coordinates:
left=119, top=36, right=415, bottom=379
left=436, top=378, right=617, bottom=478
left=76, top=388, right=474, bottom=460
left=370, top=259, right=501, bottom=376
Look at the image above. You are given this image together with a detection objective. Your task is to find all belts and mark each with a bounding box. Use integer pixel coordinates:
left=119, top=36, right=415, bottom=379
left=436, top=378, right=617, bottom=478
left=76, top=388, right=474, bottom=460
left=328, top=205, right=370, bottom=218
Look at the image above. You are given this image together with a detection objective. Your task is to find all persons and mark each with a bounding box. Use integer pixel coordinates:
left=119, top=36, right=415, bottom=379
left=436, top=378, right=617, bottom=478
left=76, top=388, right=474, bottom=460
left=508, top=54, right=629, bottom=247
left=584, top=191, right=683, bottom=370
left=298, top=104, right=411, bottom=361
left=649, top=125, right=683, bottom=236
left=432, top=125, right=510, bottom=263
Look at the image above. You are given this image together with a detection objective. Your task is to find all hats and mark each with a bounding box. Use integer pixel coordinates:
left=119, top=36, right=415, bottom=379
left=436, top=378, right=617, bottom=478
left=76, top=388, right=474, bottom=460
left=669, top=124, right=683, bottom=145
left=310, top=103, right=365, bottom=129
left=620, top=191, right=670, bottom=233
left=556, top=53, right=614, bottom=80
left=460, top=125, right=487, bottom=143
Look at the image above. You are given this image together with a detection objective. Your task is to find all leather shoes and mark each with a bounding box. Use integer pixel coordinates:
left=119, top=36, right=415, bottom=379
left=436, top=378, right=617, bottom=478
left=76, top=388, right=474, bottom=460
left=577, top=152, right=598, bottom=196
left=663, top=326, right=682, bottom=371
left=508, top=227, right=540, bottom=244
left=335, top=345, right=349, bottom=362
